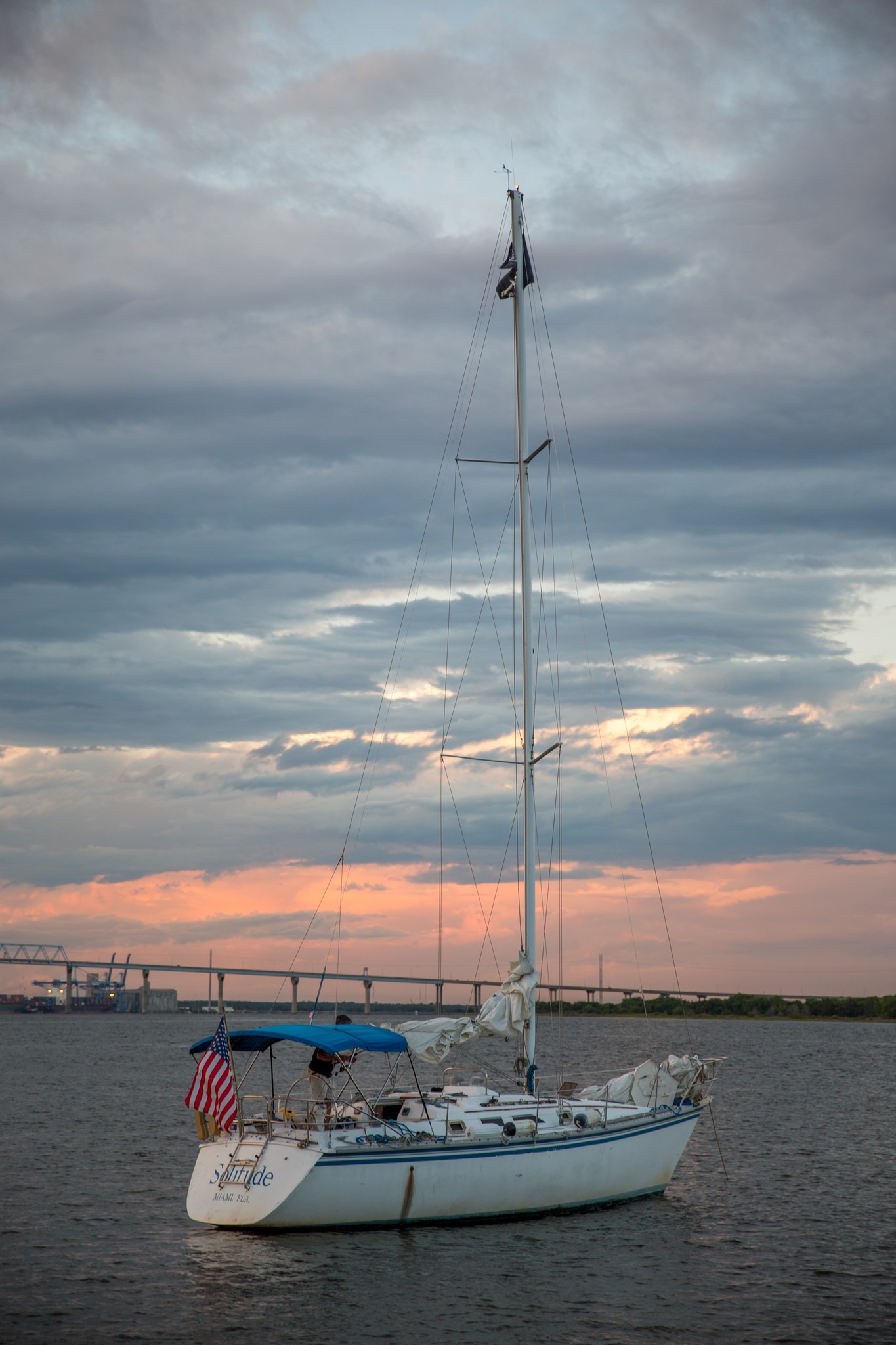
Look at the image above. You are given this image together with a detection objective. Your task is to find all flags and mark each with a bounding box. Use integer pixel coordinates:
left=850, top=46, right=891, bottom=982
left=185, top=1015, right=238, bottom=1134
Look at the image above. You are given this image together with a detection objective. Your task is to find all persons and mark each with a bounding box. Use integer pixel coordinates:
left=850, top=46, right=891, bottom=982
left=308, top=1015, right=357, bottom=1130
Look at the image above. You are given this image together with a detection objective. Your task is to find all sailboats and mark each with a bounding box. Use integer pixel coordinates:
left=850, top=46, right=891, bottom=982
left=186, top=180, right=731, bottom=1232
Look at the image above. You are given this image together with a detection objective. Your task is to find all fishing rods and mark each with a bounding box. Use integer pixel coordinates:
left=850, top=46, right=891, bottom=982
left=312, top=1020, right=433, bottom=1076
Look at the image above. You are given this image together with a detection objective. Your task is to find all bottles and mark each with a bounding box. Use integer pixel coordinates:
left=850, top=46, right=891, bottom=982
left=250, top=1115, right=255, bottom=1123
left=229, top=1118, right=237, bottom=1132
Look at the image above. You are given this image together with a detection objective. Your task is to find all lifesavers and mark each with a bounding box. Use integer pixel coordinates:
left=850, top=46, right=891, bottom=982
left=194, top=1109, right=221, bottom=1140
